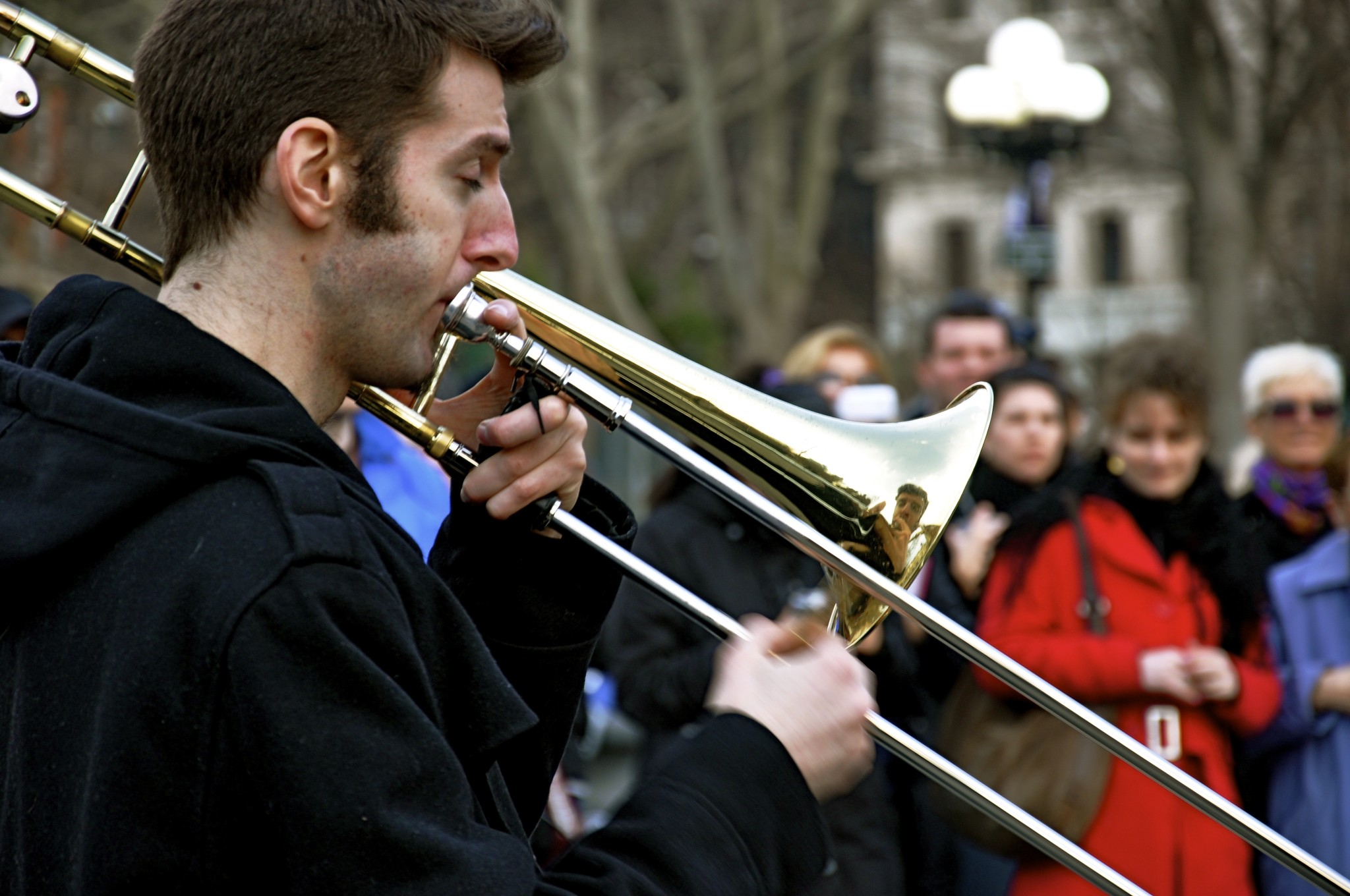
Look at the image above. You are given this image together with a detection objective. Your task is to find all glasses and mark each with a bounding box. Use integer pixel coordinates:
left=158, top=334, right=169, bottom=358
left=1113, top=425, right=1197, bottom=445
left=1252, top=398, right=1342, bottom=426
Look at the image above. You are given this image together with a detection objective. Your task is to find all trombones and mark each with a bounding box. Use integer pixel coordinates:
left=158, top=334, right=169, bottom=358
left=0, top=0, right=1347, bottom=896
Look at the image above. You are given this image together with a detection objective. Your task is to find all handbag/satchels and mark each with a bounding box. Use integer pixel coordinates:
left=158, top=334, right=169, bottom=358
left=932, top=657, right=1115, bottom=868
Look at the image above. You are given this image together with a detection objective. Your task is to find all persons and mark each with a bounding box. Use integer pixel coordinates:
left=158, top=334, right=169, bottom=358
left=925, top=367, right=1074, bottom=895
left=1234, top=343, right=1343, bottom=613
left=1258, top=432, right=1350, bottom=896
left=614, top=321, right=920, bottom=895
left=971, top=335, right=1280, bottom=895
left=900, top=295, right=1037, bottom=416
left=319, top=391, right=607, bottom=841
left=0, top=0, right=878, bottom=895
left=866, top=484, right=940, bottom=582
